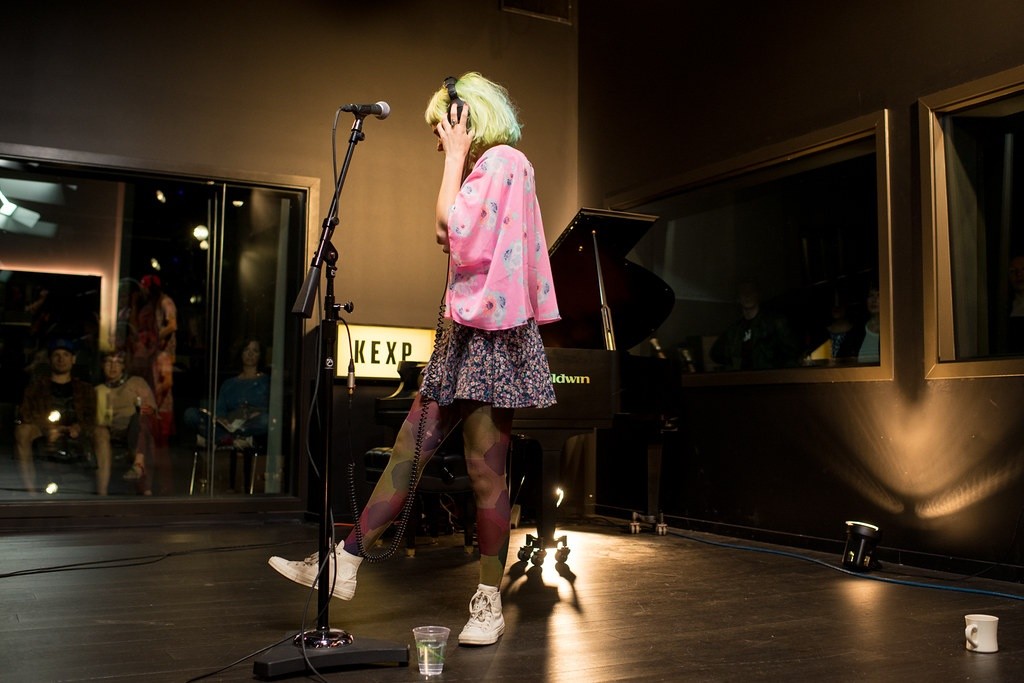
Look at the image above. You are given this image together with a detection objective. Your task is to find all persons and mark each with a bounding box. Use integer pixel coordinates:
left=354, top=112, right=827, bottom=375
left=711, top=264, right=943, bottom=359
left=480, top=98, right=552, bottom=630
left=270, top=72, right=562, bottom=645
left=15, top=274, right=266, bottom=497
left=711, top=257, right=1024, bottom=371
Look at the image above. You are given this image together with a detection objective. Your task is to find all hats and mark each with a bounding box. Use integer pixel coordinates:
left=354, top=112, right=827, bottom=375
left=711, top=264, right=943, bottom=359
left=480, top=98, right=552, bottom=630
left=140, top=275, right=161, bottom=290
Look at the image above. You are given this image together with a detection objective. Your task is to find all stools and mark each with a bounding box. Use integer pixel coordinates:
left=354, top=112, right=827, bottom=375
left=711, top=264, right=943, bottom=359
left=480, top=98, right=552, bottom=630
left=365, top=447, right=477, bottom=560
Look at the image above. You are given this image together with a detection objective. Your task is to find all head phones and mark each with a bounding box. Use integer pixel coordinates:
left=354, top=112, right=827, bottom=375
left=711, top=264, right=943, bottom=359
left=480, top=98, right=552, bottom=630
left=443, top=75, right=470, bottom=125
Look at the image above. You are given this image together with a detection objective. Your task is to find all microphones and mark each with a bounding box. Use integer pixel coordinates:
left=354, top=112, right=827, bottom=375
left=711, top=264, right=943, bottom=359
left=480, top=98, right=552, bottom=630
left=340, top=100, right=392, bottom=121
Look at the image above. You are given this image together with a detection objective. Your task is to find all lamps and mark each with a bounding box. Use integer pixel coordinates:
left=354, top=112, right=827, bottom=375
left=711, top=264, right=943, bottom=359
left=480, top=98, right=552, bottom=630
left=840, top=519, right=881, bottom=572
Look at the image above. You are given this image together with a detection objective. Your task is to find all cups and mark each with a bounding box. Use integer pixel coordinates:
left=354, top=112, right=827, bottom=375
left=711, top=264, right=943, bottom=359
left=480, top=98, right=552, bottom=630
left=965, top=614, right=999, bottom=653
left=413, top=625, right=450, bottom=676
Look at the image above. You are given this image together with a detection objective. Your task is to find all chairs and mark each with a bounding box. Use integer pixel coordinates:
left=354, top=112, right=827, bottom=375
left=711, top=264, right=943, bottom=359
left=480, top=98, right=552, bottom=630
left=32, top=406, right=260, bottom=497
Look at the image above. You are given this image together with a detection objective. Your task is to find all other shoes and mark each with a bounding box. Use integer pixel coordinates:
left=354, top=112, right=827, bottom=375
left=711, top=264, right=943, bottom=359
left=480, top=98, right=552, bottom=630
left=232, top=434, right=256, bottom=448
left=121, top=463, right=145, bottom=481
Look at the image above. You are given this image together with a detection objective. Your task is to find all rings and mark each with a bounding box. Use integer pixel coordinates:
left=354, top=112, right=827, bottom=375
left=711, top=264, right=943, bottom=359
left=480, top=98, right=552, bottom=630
left=450, top=119, right=458, bottom=128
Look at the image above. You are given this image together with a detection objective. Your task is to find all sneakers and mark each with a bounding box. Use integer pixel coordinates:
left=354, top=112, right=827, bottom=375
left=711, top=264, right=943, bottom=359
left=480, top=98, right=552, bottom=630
left=458, top=583, right=505, bottom=645
left=269, top=539, right=364, bottom=601
